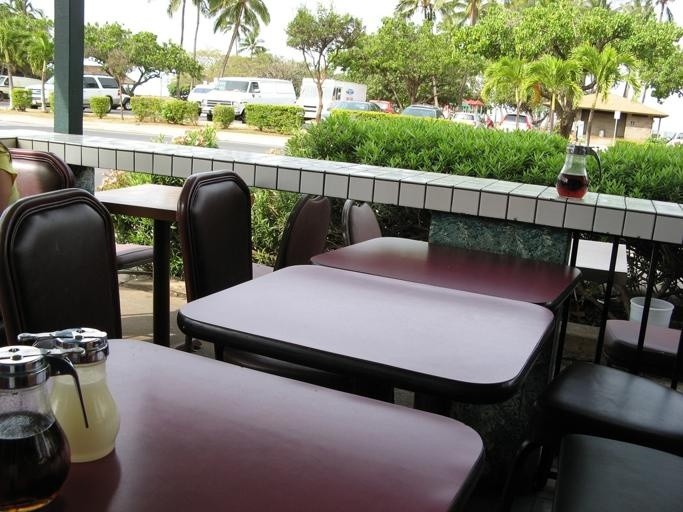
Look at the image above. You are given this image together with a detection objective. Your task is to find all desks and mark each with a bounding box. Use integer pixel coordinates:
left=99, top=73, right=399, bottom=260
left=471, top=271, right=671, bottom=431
left=1, top=338, right=484, bottom=511
left=176, top=264, right=554, bottom=411
left=311, top=236, right=582, bottom=313
left=96, top=182, right=184, bottom=348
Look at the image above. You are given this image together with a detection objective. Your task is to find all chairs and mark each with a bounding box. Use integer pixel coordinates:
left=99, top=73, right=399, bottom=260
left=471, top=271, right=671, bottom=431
left=542, top=358, right=683, bottom=450
left=552, top=433, right=682, bottom=510
left=0, top=188, right=123, bottom=348
left=177, top=169, right=350, bottom=388
left=341, top=197, right=382, bottom=246
left=8, top=149, right=73, bottom=202
left=116, top=240, right=154, bottom=272
left=602, top=317, right=682, bottom=377
left=251, top=195, right=330, bottom=280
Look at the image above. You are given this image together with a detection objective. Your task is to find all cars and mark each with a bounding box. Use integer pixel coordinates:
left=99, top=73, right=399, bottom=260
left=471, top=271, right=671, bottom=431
left=0, top=75, right=132, bottom=112
left=666, top=132, right=683, bottom=147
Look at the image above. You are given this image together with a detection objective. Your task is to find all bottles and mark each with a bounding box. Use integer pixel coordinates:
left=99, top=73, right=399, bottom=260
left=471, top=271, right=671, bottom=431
left=0, top=345, right=89, bottom=512
left=17, top=328, right=120, bottom=463
left=556, top=144, right=601, bottom=200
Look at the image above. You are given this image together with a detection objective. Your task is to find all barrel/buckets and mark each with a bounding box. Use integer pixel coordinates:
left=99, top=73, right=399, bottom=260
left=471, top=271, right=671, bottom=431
left=630, top=297, right=674, bottom=326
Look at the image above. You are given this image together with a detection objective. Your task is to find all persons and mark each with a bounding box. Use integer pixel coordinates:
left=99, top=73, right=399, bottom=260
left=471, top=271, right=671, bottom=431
left=0, top=141, right=20, bottom=217
